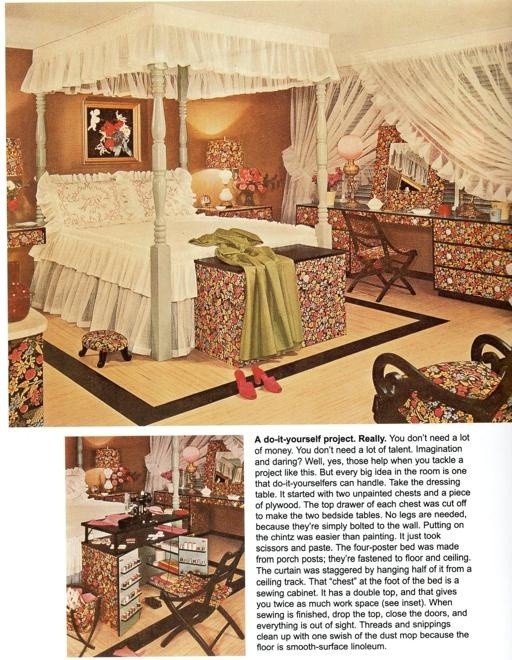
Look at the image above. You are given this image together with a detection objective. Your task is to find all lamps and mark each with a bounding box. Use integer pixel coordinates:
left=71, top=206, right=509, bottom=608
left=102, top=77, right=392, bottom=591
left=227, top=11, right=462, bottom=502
left=338, top=135, right=363, bottom=208
left=95, top=445, right=120, bottom=493
left=182, top=447, right=201, bottom=494
left=6, top=137, right=24, bottom=177
left=205, top=137, right=244, bottom=208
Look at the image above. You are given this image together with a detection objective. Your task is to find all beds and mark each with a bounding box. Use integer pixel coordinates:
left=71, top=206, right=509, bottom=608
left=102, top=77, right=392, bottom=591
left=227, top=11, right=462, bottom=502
left=28, top=167, right=319, bottom=357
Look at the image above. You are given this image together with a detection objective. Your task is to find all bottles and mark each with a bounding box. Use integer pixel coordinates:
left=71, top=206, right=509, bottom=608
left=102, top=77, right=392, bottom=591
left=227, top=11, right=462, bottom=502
left=177, top=539, right=207, bottom=578
left=120, top=559, right=142, bottom=620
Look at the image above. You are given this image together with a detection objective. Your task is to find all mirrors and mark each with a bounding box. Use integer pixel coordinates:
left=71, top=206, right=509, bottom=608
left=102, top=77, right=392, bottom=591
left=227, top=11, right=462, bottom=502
left=215, top=451, right=243, bottom=484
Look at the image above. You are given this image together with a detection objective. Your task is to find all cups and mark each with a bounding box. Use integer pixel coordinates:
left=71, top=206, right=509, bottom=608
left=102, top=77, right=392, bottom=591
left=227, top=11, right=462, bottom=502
left=487, top=208, right=501, bottom=223
left=490, top=201, right=510, bottom=222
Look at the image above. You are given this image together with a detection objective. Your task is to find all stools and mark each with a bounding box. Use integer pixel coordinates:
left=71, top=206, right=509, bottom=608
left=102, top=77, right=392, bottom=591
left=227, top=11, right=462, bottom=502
left=78, top=330, right=132, bottom=367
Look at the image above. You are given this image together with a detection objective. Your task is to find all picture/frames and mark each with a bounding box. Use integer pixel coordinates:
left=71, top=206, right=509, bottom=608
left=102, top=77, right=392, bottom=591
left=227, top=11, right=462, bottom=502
left=82, top=99, right=142, bottom=165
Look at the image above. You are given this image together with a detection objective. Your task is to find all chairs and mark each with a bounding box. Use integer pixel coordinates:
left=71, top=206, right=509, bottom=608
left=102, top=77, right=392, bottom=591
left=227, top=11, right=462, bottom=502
left=342, top=210, right=418, bottom=302
left=372, top=335, right=512, bottom=423
left=146, top=544, right=244, bottom=656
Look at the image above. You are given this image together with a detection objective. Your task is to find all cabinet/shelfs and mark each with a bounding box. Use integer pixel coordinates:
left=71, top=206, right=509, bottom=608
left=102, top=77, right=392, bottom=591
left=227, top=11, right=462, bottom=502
left=89, top=492, right=138, bottom=502
left=82, top=509, right=195, bottom=638
left=154, top=488, right=244, bottom=535
left=9, top=308, right=47, bottom=426
left=194, top=244, right=346, bottom=368
left=296, top=203, right=512, bottom=311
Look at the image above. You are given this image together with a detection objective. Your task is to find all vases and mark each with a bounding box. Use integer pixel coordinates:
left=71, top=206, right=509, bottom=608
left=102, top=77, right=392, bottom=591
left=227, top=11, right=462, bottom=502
left=8, top=262, right=31, bottom=322
left=117, top=482, right=123, bottom=492
left=242, top=191, right=256, bottom=206
left=327, top=192, right=336, bottom=208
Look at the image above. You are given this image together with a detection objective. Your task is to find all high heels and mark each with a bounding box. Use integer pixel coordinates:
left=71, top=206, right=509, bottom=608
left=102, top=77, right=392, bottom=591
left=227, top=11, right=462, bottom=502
left=235, top=370, right=257, bottom=399
left=253, top=367, right=282, bottom=393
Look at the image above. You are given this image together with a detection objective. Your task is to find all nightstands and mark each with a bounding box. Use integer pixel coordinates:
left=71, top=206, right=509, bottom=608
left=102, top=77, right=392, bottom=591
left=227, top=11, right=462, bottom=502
left=196, top=205, right=273, bottom=221
left=8, top=225, right=46, bottom=250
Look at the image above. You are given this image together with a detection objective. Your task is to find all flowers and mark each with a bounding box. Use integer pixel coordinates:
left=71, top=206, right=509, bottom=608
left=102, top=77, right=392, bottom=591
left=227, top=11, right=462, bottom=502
left=112, top=467, right=141, bottom=486
left=161, top=470, right=172, bottom=481
left=232, top=167, right=280, bottom=193
left=312, top=167, right=343, bottom=192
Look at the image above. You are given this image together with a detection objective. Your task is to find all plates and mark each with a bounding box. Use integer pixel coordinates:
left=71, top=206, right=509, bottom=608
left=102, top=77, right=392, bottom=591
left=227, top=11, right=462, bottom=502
left=411, top=207, right=433, bottom=216
left=215, top=205, right=227, bottom=211
